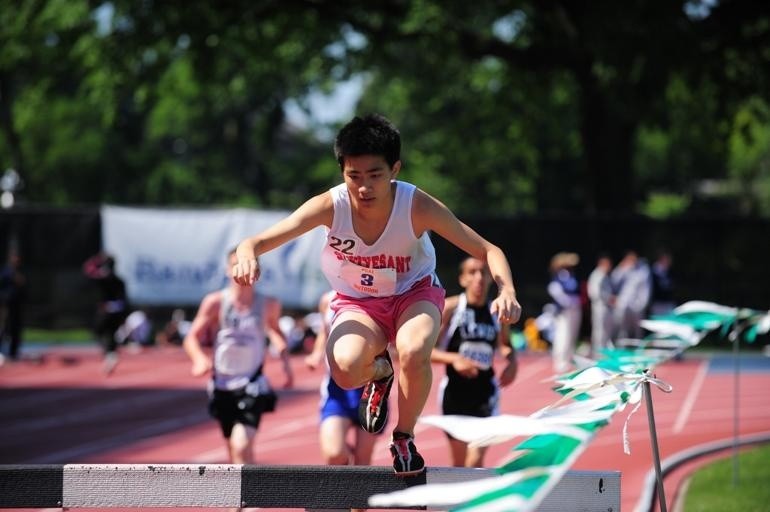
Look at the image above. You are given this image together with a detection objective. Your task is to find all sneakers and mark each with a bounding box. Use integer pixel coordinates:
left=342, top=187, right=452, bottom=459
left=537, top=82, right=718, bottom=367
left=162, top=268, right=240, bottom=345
left=390, top=425, right=425, bottom=477
left=357, top=349, right=394, bottom=435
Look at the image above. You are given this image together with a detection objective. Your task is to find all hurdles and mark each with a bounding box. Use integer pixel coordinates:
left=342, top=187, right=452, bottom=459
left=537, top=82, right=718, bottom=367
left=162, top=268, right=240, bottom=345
left=0, top=463, right=622, bottom=512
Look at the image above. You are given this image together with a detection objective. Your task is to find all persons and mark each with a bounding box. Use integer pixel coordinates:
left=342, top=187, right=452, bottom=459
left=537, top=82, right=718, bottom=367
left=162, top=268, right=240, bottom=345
left=304, top=288, right=376, bottom=511
left=1, top=242, right=28, bottom=361
left=430, top=254, right=518, bottom=468
left=547, top=249, right=676, bottom=373
left=93, top=257, right=142, bottom=377
left=183, top=247, right=288, bottom=468
left=230, top=112, right=521, bottom=477
